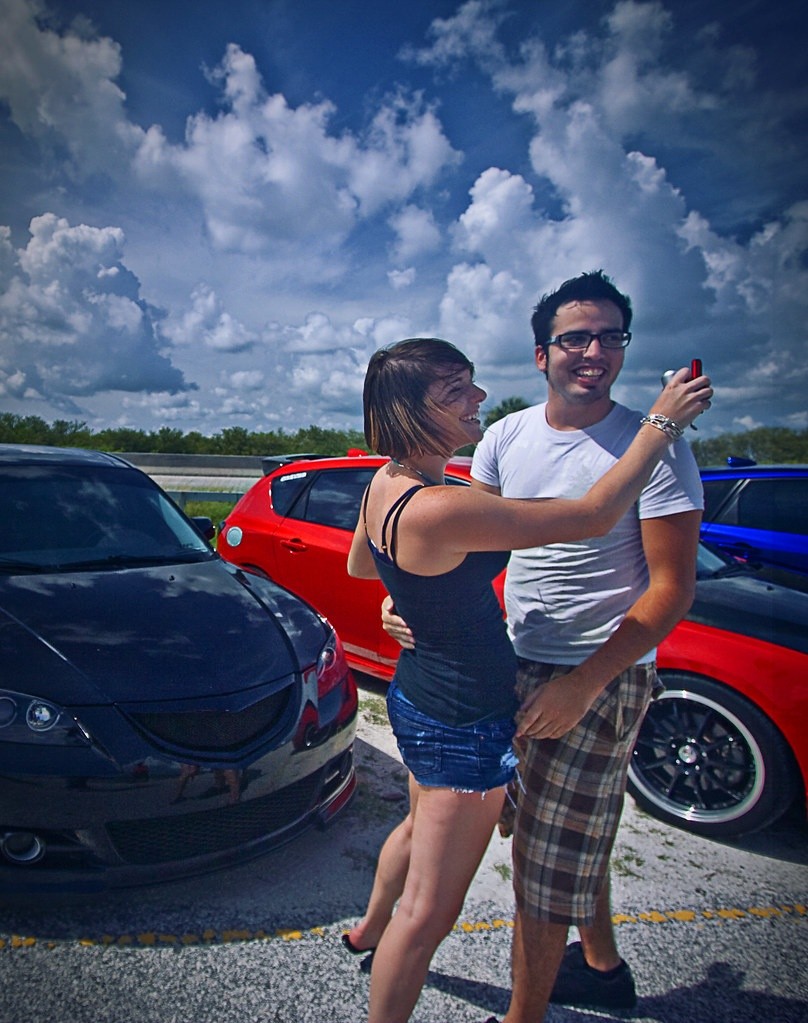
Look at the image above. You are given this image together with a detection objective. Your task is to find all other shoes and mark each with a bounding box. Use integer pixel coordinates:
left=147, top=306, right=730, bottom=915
left=548, top=942, right=636, bottom=1008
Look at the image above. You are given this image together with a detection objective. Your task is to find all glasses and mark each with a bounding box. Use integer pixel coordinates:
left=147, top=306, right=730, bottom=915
left=544, top=329, right=632, bottom=350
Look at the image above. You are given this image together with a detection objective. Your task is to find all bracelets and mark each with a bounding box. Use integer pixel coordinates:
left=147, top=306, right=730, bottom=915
left=640, top=414, right=683, bottom=441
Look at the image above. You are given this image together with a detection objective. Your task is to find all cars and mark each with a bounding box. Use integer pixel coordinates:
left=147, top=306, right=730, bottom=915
left=0, top=442, right=359, bottom=901
left=698, top=454, right=808, bottom=594
left=216, top=448, right=808, bottom=844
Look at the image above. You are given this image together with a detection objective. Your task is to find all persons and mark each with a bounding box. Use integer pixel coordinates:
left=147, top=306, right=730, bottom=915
left=341, top=338, right=712, bottom=1023
left=380, top=270, right=704, bottom=1023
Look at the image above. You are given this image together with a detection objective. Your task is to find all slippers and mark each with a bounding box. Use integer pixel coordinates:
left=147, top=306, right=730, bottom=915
left=340, top=934, right=378, bottom=954
